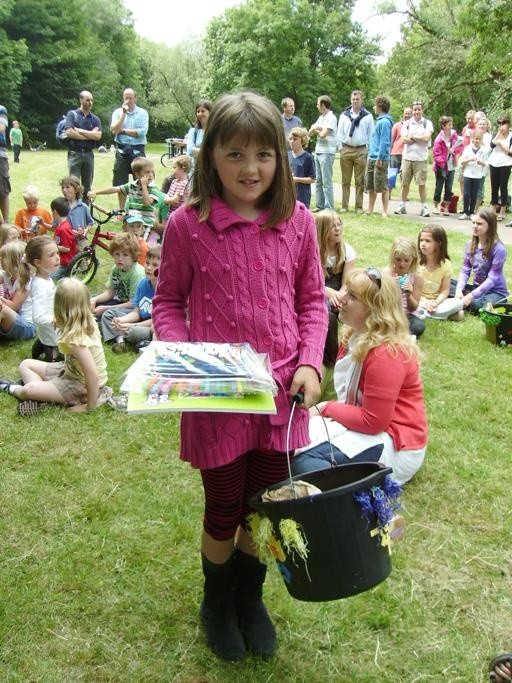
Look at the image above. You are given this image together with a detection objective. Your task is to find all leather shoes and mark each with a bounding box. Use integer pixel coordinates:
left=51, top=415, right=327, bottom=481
left=0, top=378, right=25, bottom=394
left=17, top=399, right=48, bottom=419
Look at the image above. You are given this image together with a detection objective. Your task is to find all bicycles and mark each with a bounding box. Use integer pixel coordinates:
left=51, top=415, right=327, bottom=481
left=160, top=138, right=189, bottom=169
left=65, top=200, right=125, bottom=284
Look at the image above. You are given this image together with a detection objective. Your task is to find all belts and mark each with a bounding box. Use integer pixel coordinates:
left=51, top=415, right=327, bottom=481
left=342, top=144, right=366, bottom=149
left=71, top=148, right=92, bottom=152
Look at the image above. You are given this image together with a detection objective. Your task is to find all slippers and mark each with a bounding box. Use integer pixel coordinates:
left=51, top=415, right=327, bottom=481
left=497, top=215, right=506, bottom=221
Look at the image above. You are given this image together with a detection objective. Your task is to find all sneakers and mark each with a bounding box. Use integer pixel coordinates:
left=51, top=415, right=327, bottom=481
left=450, top=310, right=465, bottom=322
left=393, top=205, right=407, bottom=215
left=458, top=213, right=470, bottom=220
left=469, top=215, right=475, bottom=220
left=420, top=209, right=430, bottom=216
left=111, top=341, right=127, bottom=354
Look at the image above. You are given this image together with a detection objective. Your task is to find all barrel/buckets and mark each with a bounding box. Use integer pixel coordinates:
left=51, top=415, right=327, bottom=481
left=482, top=294, right=512, bottom=347
left=246, top=391, right=394, bottom=605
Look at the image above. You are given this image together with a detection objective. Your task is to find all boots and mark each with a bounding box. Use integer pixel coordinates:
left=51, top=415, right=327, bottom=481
left=200, top=569, right=247, bottom=661
left=235, top=564, right=278, bottom=659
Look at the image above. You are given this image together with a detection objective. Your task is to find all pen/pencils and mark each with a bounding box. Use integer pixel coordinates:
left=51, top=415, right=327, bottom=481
left=146, top=379, right=249, bottom=400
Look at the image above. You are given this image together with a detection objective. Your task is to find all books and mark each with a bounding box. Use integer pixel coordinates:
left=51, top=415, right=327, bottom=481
left=128, top=362, right=280, bottom=415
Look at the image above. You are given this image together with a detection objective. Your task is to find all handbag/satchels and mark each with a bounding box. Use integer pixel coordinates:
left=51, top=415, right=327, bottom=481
left=56, top=110, right=79, bottom=144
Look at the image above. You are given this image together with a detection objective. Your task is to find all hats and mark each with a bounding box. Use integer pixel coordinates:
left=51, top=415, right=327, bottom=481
left=122, top=210, right=147, bottom=224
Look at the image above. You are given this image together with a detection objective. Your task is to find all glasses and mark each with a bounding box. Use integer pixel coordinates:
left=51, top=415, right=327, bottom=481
left=366, top=266, right=384, bottom=288
left=412, top=100, right=423, bottom=105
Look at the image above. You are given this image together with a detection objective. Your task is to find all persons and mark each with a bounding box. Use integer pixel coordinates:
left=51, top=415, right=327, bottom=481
left=164, top=154, right=193, bottom=214
left=315, top=210, right=357, bottom=364
left=293, top=269, right=428, bottom=489
left=150, top=92, right=329, bottom=662
left=454, top=208, right=508, bottom=310
left=380, top=239, right=428, bottom=340
left=281, top=90, right=511, bottom=227
left=417, top=227, right=465, bottom=322
left=187, top=100, right=213, bottom=175
